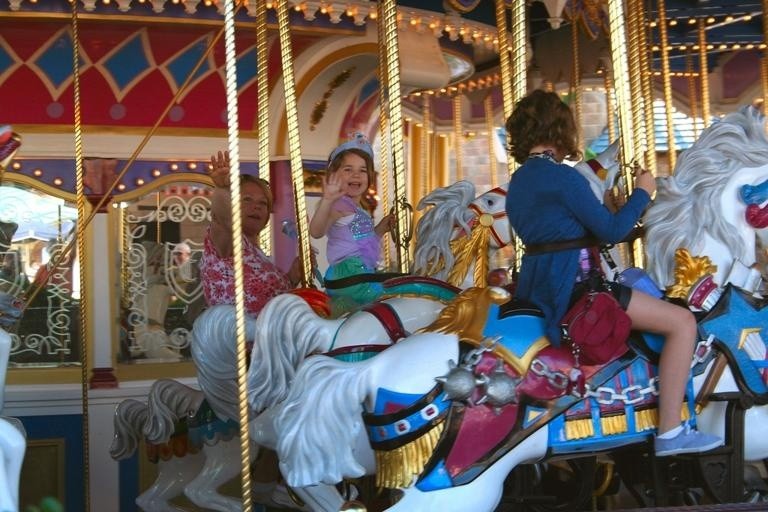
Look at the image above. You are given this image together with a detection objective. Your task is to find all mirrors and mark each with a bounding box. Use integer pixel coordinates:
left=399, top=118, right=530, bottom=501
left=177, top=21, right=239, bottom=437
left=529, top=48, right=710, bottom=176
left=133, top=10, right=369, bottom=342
left=1, top=172, right=96, bottom=383
left=105, top=172, right=217, bottom=382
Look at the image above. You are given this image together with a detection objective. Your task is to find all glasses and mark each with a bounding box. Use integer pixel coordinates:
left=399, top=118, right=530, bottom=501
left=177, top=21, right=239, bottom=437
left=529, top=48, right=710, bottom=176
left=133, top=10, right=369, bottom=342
left=238, top=174, right=269, bottom=190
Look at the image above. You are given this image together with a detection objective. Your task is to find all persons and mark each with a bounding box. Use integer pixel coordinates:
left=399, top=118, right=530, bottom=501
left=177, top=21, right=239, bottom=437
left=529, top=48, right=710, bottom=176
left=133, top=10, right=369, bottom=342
left=504, top=89, right=725, bottom=458
left=310, top=138, right=396, bottom=302
left=164, top=244, right=208, bottom=358
left=199, top=150, right=317, bottom=368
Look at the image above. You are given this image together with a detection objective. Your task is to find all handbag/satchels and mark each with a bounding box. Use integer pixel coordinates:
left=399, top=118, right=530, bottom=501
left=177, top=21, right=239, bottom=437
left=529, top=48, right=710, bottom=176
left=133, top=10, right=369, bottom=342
left=557, top=292, right=632, bottom=366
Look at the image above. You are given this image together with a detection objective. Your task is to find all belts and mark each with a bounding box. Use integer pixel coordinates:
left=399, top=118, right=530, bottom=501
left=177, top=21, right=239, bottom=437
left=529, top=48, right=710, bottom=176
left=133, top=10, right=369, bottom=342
left=522, top=239, right=589, bottom=254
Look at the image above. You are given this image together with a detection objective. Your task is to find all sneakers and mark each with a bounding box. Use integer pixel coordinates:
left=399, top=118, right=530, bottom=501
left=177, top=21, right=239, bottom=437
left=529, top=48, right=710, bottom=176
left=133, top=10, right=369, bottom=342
left=652, top=421, right=722, bottom=456
